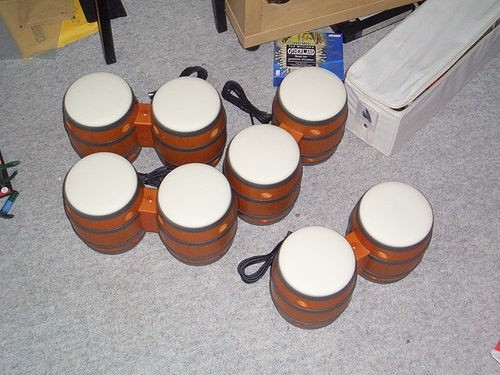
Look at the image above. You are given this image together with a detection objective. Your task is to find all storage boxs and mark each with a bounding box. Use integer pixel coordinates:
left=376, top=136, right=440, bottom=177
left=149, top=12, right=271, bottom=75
left=343, top=0, right=500, bottom=157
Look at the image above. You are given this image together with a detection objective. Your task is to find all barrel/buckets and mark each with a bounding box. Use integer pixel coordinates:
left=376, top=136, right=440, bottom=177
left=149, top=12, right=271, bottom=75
left=62, top=151, right=145, bottom=255
left=221, top=124, right=302, bottom=225
left=155, top=162, right=236, bottom=266
left=346, top=181, right=434, bottom=284
left=150, top=76, right=227, bottom=170
left=270, top=226, right=359, bottom=329
left=272, top=66, right=348, bottom=166
left=61, top=71, right=141, bottom=163
